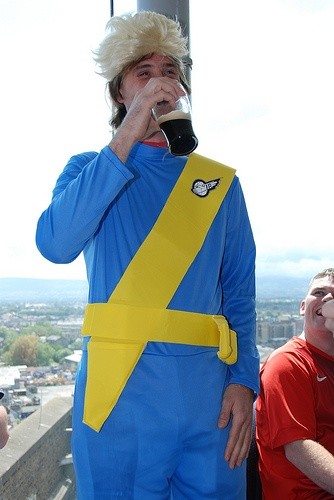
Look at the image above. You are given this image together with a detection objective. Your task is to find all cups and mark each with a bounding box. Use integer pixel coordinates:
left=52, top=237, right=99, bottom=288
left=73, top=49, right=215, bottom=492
left=151, top=80, right=199, bottom=158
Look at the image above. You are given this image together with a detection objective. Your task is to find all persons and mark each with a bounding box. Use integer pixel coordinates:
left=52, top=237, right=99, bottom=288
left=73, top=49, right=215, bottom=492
left=254, top=267, right=334, bottom=500
left=0, top=391, right=10, bottom=452
left=35, top=8, right=263, bottom=500
